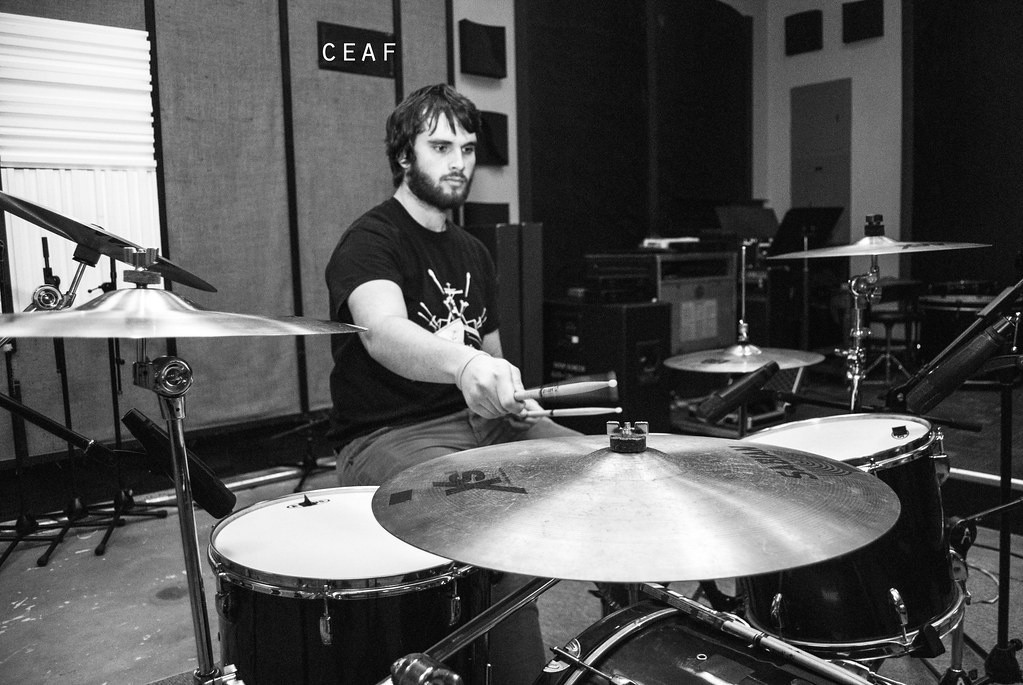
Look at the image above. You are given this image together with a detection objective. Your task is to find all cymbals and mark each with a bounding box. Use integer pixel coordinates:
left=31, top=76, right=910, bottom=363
left=0, top=288, right=369, bottom=340
left=0, top=190, right=220, bottom=294
left=663, top=343, right=826, bottom=374
left=766, top=235, right=993, bottom=261
left=370, top=431, right=901, bottom=585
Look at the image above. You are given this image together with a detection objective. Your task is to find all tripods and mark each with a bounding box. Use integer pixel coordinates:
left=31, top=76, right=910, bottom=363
left=0, top=238, right=177, bottom=570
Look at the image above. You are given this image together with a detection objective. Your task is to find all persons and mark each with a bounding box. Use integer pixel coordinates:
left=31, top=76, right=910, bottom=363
left=322, top=84, right=665, bottom=685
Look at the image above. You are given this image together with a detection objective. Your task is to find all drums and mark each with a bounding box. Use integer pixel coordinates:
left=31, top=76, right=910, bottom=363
left=735, top=411, right=965, bottom=662
left=205, top=483, right=495, bottom=685
left=530, top=598, right=908, bottom=685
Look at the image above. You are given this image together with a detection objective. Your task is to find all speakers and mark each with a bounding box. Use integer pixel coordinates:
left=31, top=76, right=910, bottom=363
left=542, top=297, right=673, bottom=435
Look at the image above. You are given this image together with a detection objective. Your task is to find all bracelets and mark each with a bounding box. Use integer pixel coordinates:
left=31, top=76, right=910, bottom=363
left=458, top=352, right=491, bottom=390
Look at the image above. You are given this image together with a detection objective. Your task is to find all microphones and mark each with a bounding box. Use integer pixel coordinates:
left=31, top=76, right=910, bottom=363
left=699, top=361, right=780, bottom=425
left=905, top=315, right=1017, bottom=416
left=121, top=409, right=236, bottom=521
